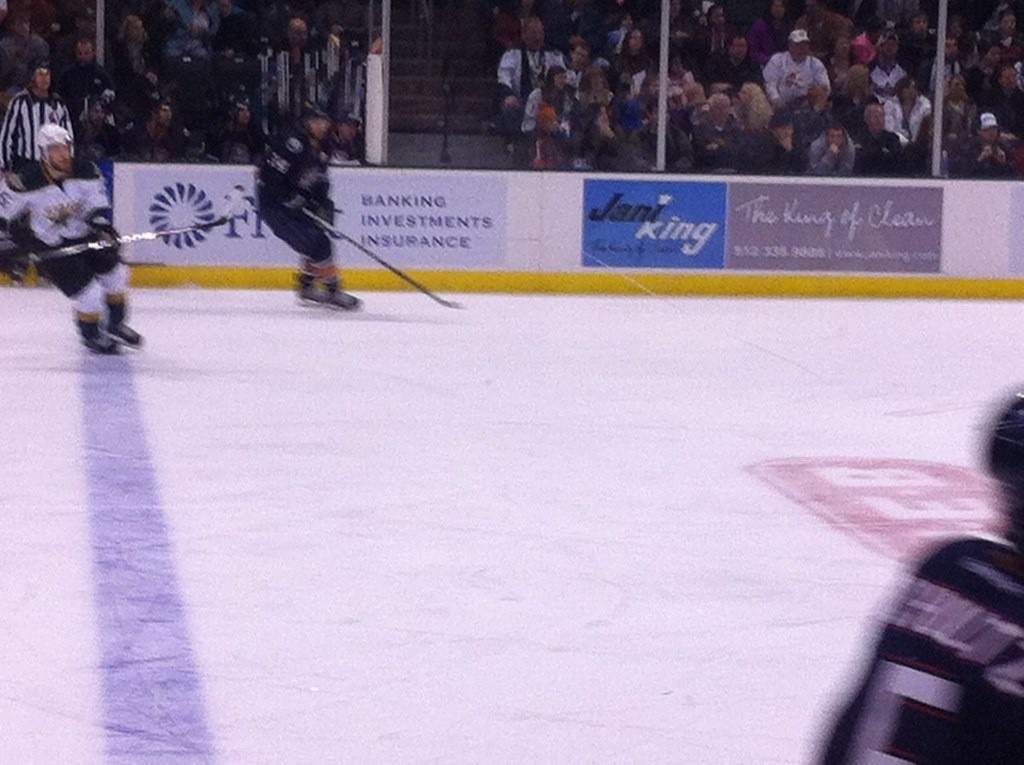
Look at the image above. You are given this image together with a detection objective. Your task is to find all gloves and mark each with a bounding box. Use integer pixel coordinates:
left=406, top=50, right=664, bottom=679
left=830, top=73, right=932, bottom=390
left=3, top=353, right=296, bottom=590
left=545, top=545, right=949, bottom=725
left=86, top=212, right=122, bottom=253
left=277, top=179, right=316, bottom=210
left=0, top=229, right=45, bottom=282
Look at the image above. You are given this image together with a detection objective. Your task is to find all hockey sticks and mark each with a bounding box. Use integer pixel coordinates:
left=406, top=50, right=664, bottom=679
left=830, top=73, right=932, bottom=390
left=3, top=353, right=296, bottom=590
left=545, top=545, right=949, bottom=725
left=301, top=206, right=473, bottom=312
left=25, top=184, right=248, bottom=263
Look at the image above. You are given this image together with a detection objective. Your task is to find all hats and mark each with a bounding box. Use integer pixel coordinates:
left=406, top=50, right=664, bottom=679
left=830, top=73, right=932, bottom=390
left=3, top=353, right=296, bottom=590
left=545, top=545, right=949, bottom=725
left=788, top=29, right=811, bottom=44
left=977, top=112, right=1001, bottom=131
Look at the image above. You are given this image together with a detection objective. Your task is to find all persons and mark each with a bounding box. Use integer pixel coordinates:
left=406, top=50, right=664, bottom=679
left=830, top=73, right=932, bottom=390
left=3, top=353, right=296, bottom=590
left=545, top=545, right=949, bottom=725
left=0, top=60, right=77, bottom=286
left=818, top=389, right=1024, bottom=764
left=477, top=0, right=1024, bottom=178
left=0, top=0, right=381, bottom=169
left=256, top=102, right=362, bottom=312
left=4, top=122, right=142, bottom=354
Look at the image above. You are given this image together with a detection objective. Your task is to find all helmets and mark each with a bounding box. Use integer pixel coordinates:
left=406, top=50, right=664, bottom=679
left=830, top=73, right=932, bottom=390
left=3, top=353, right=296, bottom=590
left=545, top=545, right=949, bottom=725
left=303, top=103, right=334, bottom=126
left=26, top=57, right=53, bottom=80
left=331, top=110, right=362, bottom=129
left=36, top=122, right=75, bottom=166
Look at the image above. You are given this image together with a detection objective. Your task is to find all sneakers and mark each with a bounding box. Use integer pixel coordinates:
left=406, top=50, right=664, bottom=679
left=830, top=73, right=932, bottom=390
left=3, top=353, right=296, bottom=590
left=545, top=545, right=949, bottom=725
left=325, top=287, right=361, bottom=310
left=105, top=320, right=140, bottom=350
left=295, top=285, right=332, bottom=313
left=82, top=332, right=121, bottom=358
